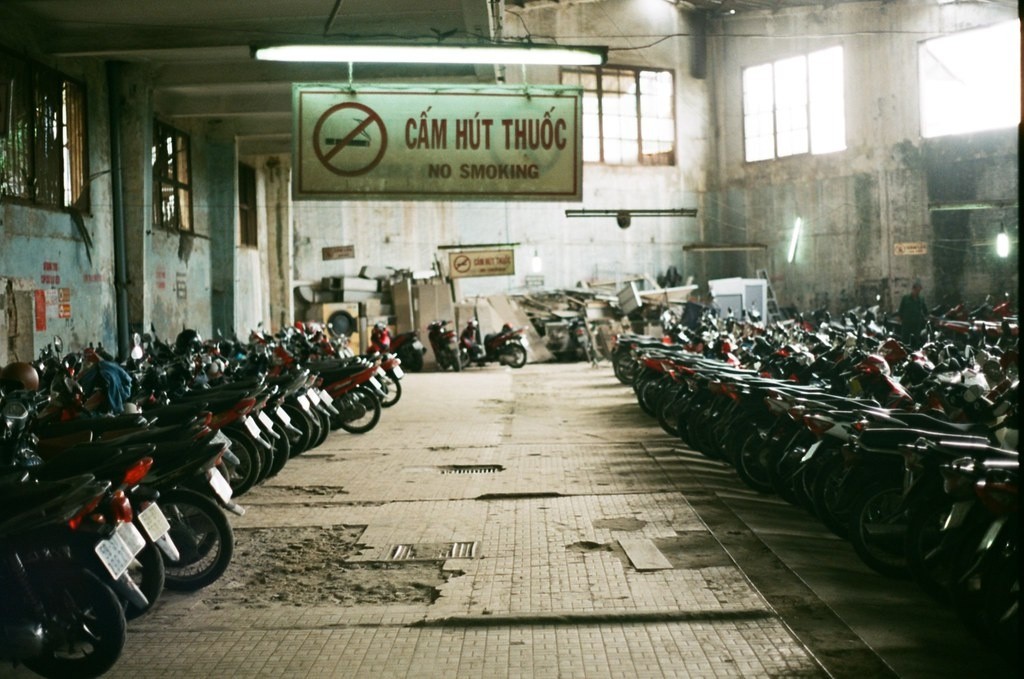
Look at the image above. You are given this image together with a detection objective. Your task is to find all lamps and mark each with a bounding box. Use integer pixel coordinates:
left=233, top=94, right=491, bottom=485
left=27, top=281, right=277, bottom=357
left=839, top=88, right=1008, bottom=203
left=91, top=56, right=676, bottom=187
left=997, top=222, right=1009, bottom=259
left=682, top=244, right=767, bottom=252
left=248, top=40, right=608, bottom=67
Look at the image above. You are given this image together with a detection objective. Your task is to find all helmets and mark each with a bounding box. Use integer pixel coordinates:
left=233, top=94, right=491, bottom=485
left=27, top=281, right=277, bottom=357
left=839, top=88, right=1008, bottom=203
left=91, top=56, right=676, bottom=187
left=178, top=329, right=203, bottom=353
left=1, top=362, right=39, bottom=394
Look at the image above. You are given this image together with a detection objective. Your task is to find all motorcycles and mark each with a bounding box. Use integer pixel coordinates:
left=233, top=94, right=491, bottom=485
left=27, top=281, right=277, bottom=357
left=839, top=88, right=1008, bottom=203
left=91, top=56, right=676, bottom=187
left=611, top=298, right=1024, bottom=634
left=0, top=312, right=429, bottom=678
left=427, top=320, right=462, bottom=373
left=455, top=320, right=528, bottom=369
left=551, top=301, right=596, bottom=363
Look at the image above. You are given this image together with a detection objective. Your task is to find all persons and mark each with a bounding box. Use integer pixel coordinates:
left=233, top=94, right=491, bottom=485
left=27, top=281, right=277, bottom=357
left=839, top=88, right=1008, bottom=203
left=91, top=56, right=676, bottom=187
left=898, top=282, right=931, bottom=350
